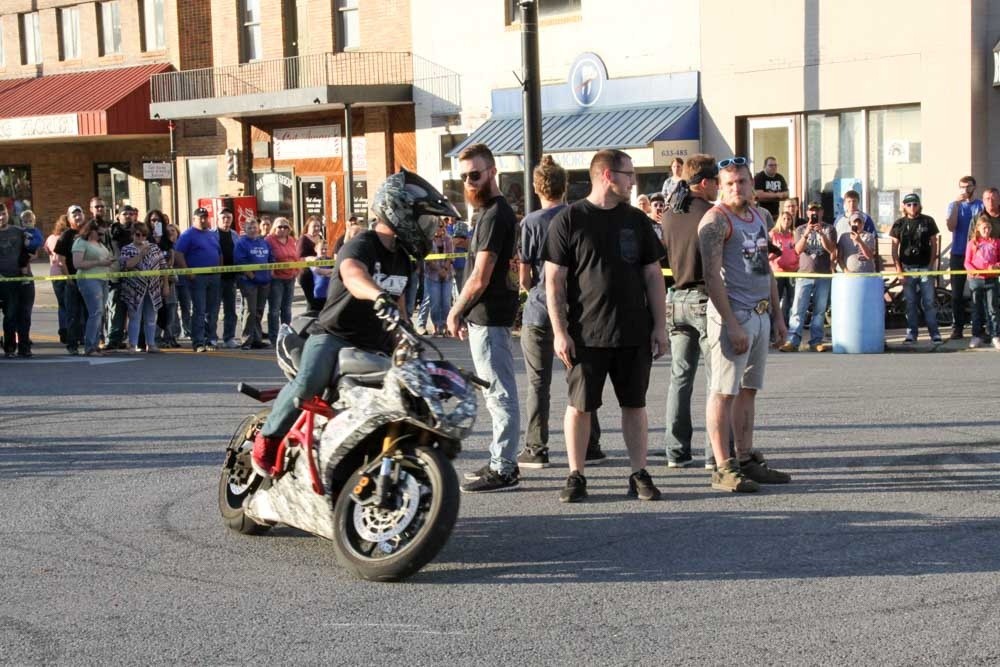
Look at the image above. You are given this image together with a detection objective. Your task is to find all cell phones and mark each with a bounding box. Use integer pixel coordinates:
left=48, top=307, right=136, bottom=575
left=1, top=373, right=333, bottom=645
left=657, top=208, right=663, bottom=215
left=852, top=225, right=857, bottom=233
left=811, top=214, right=818, bottom=224
left=154, top=222, right=162, bottom=236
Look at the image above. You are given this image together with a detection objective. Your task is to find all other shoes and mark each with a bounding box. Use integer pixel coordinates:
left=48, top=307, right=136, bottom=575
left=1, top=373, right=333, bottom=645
left=705, top=457, right=715, bottom=469
left=779, top=343, right=828, bottom=353
left=418, top=326, right=428, bottom=336
left=668, top=455, right=691, bottom=466
left=5, top=333, right=279, bottom=358
left=903, top=327, right=1000, bottom=349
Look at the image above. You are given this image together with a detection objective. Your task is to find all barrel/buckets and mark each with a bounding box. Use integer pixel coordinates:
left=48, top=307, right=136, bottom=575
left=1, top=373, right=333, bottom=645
left=831, top=275, right=884, bottom=356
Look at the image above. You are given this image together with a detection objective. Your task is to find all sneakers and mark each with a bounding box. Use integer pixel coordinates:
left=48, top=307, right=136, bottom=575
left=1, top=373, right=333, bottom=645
left=514, top=449, right=549, bottom=468
left=250, top=431, right=278, bottom=479
left=559, top=470, right=588, bottom=502
left=585, top=447, right=607, bottom=465
left=713, top=453, right=791, bottom=492
left=459, top=469, right=521, bottom=494
left=626, top=469, right=662, bottom=499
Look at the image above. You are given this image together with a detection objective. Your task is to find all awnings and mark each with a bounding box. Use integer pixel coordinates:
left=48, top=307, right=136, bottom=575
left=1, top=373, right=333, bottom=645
left=444, top=99, right=700, bottom=157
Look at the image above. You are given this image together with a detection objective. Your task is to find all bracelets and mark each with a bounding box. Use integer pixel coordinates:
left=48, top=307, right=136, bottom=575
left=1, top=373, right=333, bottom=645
left=802, top=235, right=808, bottom=239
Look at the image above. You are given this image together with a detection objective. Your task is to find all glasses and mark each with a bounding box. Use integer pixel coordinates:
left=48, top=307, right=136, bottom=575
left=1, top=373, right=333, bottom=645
left=907, top=204, right=920, bottom=207
left=91, top=205, right=162, bottom=236
left=603, top=169, right=635, bottom=179
left=717, top=158, right=747, bottom=170
left=461, top=166, right=494, bottom=183
left=278, top=225, right=289, bottom=229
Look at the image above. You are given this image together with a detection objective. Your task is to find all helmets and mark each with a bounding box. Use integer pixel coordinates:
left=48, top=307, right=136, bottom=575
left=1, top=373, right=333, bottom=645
left=370, top=170, right=463, bottom=262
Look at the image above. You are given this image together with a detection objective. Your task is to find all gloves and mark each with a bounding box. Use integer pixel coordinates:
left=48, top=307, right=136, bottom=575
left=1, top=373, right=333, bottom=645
left=374, top=295, right=402, bottom=332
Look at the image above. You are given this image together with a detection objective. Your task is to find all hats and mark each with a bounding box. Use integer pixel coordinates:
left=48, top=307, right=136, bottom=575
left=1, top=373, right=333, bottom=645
left=195, top=208, right=208, bottom=216
left=68, top=205, right=82, bottom=214
left=220, top=207, right=233, bottom=214
left=903, top=194, right=920, bottom=204
left=850, top=211, right=864, bottom=222
left=808, top=202, right=823, bottom=210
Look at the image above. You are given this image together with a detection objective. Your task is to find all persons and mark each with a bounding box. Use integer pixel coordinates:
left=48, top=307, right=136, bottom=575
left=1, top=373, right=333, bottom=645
left=540, top=150, right=664, bottom=500
left=0, top=145, right=1000, bottom=493
